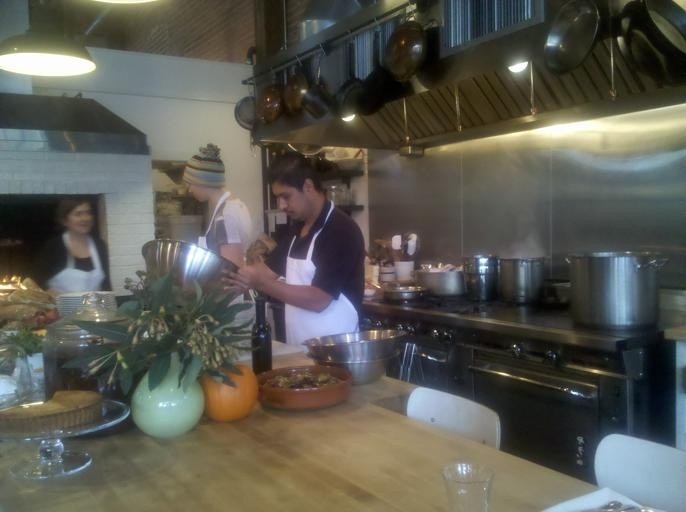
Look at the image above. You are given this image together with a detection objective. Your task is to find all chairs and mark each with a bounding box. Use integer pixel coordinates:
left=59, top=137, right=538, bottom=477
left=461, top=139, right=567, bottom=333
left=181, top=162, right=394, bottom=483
left=590, top=431, right=686, bottom=512
left=401, top=384, right=503, bottom=451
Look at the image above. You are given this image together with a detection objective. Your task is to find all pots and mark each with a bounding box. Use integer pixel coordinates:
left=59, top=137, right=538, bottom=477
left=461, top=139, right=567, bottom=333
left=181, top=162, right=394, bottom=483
left=464, top=254, right=499, bottom=303
left=616, top=0, right=686, bottom=86
left=279, top=66, right=310, bottom=118
left=301, top=52, right=331, bottom=119
left=358, top=30, right=388, bottom=116
left=384, top=15, right=425, bottom=83
left=497, top=256, right=546, bottom=304
left=333, top=42, right=364, bottom=118
left=543, top=0, right=600, bottom=74
left=563, top=251, right=670, bottom=330
left=256, top=85, right=283, bottom=123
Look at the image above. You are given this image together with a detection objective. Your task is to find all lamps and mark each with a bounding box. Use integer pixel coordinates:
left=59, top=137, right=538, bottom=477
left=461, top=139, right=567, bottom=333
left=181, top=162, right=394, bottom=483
left=0, top=1, right=97, bottom=80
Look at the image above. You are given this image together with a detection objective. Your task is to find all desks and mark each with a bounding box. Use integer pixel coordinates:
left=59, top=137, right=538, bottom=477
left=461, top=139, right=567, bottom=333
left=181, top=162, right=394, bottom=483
left=1, top=329, right=599, bottom=511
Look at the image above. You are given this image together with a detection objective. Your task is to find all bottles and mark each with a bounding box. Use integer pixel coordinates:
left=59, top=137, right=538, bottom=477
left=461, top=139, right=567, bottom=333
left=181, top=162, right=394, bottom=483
left=251, top=296, right=272, bottom=376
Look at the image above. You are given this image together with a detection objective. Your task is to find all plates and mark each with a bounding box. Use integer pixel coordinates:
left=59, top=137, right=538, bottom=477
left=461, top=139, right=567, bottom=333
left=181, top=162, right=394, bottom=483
left=55, top=290, right=119, bottom=317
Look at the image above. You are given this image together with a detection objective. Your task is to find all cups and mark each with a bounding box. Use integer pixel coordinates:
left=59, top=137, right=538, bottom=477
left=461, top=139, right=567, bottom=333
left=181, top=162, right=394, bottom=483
left=394, top=261, right=415, bottom=283
left=442, top=461, right=493, bottom=512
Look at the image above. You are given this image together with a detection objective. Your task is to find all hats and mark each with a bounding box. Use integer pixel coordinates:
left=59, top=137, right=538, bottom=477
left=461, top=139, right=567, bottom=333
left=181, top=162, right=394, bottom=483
left=183, top=143, right=227, bottom=188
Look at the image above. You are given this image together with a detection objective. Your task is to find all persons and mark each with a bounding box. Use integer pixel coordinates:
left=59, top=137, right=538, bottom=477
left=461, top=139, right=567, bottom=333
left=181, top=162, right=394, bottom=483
left=182, top=143, right=254, bottom=328
left=30, top=195, right=112, bottom=292
left=221, top=151, right=366, bottom=361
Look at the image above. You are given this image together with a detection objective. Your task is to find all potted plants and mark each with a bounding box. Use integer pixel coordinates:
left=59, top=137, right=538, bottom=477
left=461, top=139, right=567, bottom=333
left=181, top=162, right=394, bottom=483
left=1, top=276, right=56, bottom=407
left=71, top=267, right=257, bottom=436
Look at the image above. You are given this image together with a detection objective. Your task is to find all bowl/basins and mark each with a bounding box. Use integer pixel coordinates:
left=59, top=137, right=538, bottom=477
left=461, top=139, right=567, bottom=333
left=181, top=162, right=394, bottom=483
left=307, top=348, right=401, bottom=387
left=364, top=288, right=376, bottom=297
left=301, top=329, right=410, bottom=360
left=141, top=237, right=239, bottom=285
left=257, top=364, right=353, bottom=412
left=383, top=285, right=421, bottom=304
left=380, top=266, right=395, bottom=282
left=417, top=269, right=466, bottom=298
